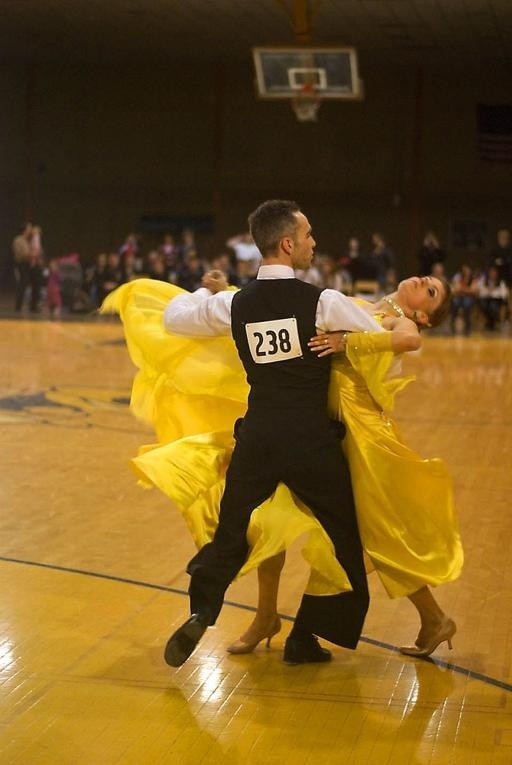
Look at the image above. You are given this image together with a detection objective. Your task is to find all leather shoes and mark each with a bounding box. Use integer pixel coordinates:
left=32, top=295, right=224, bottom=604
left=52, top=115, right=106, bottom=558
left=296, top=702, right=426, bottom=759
left=164, top=616, right=207, bottom=667
left=284, top=635, right=331, bottom=663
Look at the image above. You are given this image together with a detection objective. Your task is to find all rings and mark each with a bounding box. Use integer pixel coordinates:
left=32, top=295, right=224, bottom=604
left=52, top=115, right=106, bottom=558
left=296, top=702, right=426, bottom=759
left=324, top=339, right=328, bottom=344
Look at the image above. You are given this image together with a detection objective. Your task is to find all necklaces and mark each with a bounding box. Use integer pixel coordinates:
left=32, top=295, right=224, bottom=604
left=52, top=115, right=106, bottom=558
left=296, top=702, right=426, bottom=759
left=382, top=296, right=405, bottom=319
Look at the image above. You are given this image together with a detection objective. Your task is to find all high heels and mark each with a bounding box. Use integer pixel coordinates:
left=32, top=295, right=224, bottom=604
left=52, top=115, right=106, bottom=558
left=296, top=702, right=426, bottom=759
left=226, top=614, right=281, bottom=654
left=400, top=619, right=456, bottom=656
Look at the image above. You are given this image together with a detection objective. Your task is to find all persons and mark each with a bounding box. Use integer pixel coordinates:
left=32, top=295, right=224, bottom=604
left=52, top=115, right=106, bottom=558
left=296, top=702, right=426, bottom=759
left=13, top=223, right=263, bottom=314
left=294, top=233, right=396, bottom=303
left=422, top=229, right=511, bottom=336
left=162, top=199, right=387, bottom=669
left=98, top=269, right=465, bottom=656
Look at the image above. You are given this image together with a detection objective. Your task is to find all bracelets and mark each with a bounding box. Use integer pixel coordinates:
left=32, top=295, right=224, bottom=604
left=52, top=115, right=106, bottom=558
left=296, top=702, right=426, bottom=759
left=342, top=332, right=346, bottom=350
